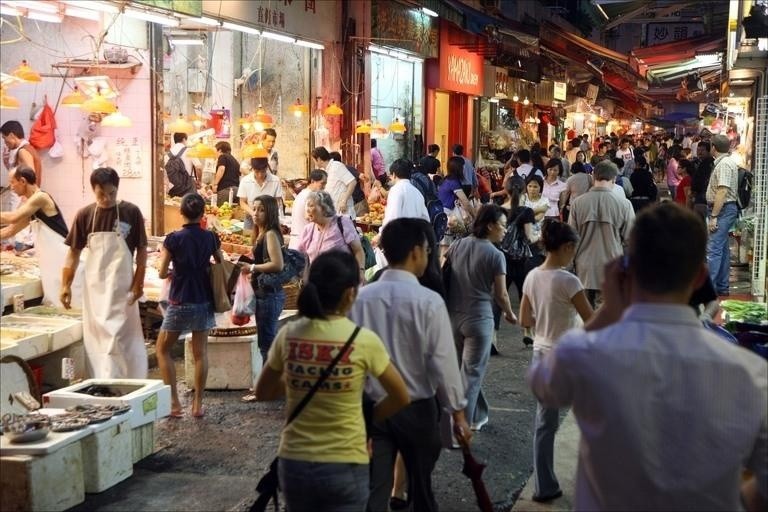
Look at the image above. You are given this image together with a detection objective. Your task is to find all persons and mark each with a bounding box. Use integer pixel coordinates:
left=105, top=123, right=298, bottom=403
left=371, top=139, right=387, bottom=185
left=624, top=147, right=652, bottom=178
left=646, top=135, right=659, bottom=174
left=529, top=147, right=546, bottom=178
left=490, top=176, right=542, bottom=358
left=61, top=167, right=147, bottom=380
left=346, top=217, right=473, bottom=512
left=0, top=121, right=41, bottom=251
left=166, top=132, right=202, bottom=199
left=211, top=142, right=240, bottom=207
left=420, top=144, right=443, bottom=178
left=364, top=220, right=446, bottom=512
left=682, top=147, right=692, bottom=160
left=236, top=158, right=283, bottom=229
left=683, top=133, right=695, bottom=149
left=441, top=203, right=518, bottom=451
left=656, top=143, right=669, bottom=183
left=286, top=169, right=327, bottom=250
left=576, top=151, right=592, bottom=176
left=0, top=164, right=85, bottom=311
left=411, top=162, right=438, bottom=200
left=689, top=141, right=712, bottom=220
left=550, top=147, right=570, bottom=183
left=637, top=138, right=648, bottom=152
left=564, top=161, right=595, bottom=212
left=238, top=195, right=284, bottom=402
left=674, top=159, right=692, bottom=210
left=452, top=144, right=478, bottom=198
left=590, top=143, right=610, bottom=167
left=438, top=157, right=478, bottom=269
left=508, top=149, right=545, bottom=182
left=599, top=136, right=604, bottom=145
left=329, top=151, right=373, bottom=216
left=519, top=217, right=593, bottom=503
left=567, top=161, right=636, bottom=308
left=540, top=149, right=550, bottom=168
left=524, top=175, right=551, bottom=254
left=240, top=129, right=279, bottom=174
left=667, top=144, right=683, bottom=200
left=527, top=201, right=768, bottom=512
left=705, top=134, right=740, bottom=298
left=630, top=154, right=657, bottom=212
left=580, top=132, right=593, bottom=152
left=504, top=150, right=514, bottom=175
left=299, top=194, right=368, bottom=287
left=660, top=132, right=676, bottom=149
left=311, top=147, right=358, bottom=223
left=253, top=248, right=411, bottom=512
left=612, top=158, right=633, bottom=198
left=490, top=159, right=519, bottom=199
left=156, top=196, right=225, bottom=419
left=605, top=139, right=615, bottom=160
left=616, top=138, right=634, bottom=173
left=691, top=135, right=702, bottom=159
left=549, top=138, right=560, bottom=152
left=564, top=138, right=582, bottom=164
left=372, top=159, right=430, bottom=249
left=542, top=158, right=566, bottom=222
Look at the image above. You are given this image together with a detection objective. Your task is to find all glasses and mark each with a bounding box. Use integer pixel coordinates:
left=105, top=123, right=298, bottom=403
left=676, top=166, right=682, bottom=169
left=419, top=247, right=432, bottom=255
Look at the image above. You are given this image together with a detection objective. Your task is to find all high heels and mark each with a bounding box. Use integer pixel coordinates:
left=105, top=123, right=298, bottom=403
left=523, top=336, right=534, bottom=348
left=491, top=344, right=500, bottom=356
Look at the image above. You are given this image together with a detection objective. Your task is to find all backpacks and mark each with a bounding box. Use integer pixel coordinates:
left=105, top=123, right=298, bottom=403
left=736, top=164, right=752, bottom=210
left=500, top=208, right=533, bottom=261
left=166, top=147, right=193, bottom=197
left=411, top=174, right=447, bottom=242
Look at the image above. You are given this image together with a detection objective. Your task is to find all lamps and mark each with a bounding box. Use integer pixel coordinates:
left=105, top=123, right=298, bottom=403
left=389, top=118, right=406, bottom=134
left=323, top=101, right=343, bottom=115
left=174, top=113, right=191, bottom=134
left=365, top=44, right=425, bottom=64
left=173, top=12, right=221, bottom=26
left=82, top=87, right=115, bottom=113
left=296, top=40, right=325, bottom=50
left=63, top=1, right=120, bottom=15
left=25, top=10, right=63, bottom=23
left=241, top=141, right=268, bottom=158
left=1, top=5, right=22, bottom=17
left=0, top=90, right=20, bottom=109
left=355, top=120, right=363, bottom=133
left=238, top=113, right=252, bottom=131
left=11, top=59, right=41, bottom=83
left=250, top=104, right=273, bottom=131
left=0, top=73, right=23, bottom=87
left=65, top=6, right=99, bottom=21
left=1, top=1, right=59, bottom=14
left=364, top=120, right=374, bottom=133
left=368, top=122, right=389, bottom=138
left=123, top=8, right=166, bottom=24
left=222, top=22, right=258, bottom=34
left=186, top=137, right=215, bottom=159
left=421, top=7, right=439, bottom=18
left=101, top=106, right=131, bottom=128
left=288, top=98, right=308, bottom=117
left=263, top=31, right=295, bottom=44
left=62, top=87, right=87, bottom=105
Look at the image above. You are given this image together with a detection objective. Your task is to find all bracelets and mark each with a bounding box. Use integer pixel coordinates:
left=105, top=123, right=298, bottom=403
left=360, top=267, right=365, bottom=271
left=250, top=264, right=255, bottom=275
left=711, top=215, right=718, bottom=218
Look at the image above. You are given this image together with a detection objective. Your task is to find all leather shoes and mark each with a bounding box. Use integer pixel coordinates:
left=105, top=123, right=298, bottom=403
left=390, top=490, right=410, bottom=512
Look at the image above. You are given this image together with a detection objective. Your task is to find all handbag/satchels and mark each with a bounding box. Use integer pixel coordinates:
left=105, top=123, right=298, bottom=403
left=207, top=231, right=241, bottom=313
left=337, top=215, right=378, bottom=273
left=495, top=206, right=534, bottom=264
left=256, top=227, right=307, bottom=296
left=247, top=324, right=360, bottom=511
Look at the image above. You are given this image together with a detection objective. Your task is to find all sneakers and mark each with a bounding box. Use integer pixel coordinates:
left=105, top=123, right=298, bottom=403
left=470, top=415, right=489, bottom=431
left=450, top=436, right=460, bottom=449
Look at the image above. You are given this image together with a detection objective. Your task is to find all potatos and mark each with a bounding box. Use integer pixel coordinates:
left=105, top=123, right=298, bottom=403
left=361, top=202, right=385, bottom=225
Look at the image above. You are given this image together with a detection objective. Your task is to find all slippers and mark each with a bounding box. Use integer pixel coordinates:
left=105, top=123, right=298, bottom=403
left=167, top=410, right=187, bottom=418
left=241, top=394, right=258, bottom=405
left=193, top=404, right=206, bottom=417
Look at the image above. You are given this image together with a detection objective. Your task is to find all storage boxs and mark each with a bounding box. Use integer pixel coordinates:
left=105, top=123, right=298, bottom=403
left=184, top=335, right=263, bottom=388
left=0, top=303, right=85, bottom=360
left=31, top=408, right=134, bottom=493
left=0, top=248, right=43, bottom=307
left=43, top=377, right=164, bottom=463
left=1, top=431, right=86, bottom=512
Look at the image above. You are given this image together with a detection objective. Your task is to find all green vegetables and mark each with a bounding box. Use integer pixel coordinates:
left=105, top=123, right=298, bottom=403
left=720, top=300, right=767, bottom=325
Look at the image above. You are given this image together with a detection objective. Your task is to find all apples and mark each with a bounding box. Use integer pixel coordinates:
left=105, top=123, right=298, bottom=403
left=221, top=202, right=230, bottom=216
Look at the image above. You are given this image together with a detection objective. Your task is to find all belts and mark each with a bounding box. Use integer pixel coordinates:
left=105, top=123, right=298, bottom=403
left=709, top=201, right=736, bottom=208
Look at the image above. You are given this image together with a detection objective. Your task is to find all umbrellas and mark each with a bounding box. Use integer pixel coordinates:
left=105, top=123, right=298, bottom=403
left=454, top=426, right=493, bottom=512
